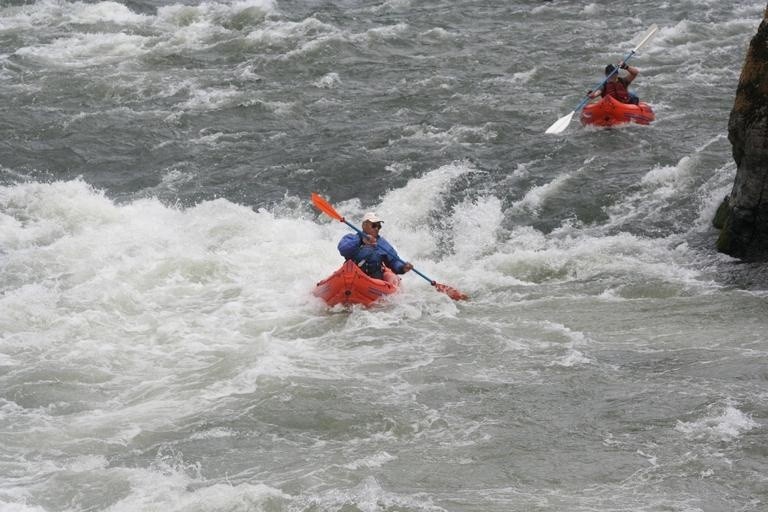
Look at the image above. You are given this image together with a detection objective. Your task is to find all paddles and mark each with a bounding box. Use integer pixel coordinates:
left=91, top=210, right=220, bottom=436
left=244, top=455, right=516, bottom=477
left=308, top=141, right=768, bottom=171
left=545, top=24, right=659, bottom=133
left=311, top=192, right=468, bottom=300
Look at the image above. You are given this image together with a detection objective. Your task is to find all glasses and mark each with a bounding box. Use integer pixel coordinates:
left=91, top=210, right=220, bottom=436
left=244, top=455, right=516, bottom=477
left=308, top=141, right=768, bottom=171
left=372, top=224, right=381, bottom=229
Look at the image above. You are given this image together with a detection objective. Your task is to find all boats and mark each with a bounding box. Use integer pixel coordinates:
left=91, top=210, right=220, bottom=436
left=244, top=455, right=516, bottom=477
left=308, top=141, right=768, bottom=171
left=310, top=258, right=404, bottom=308
left=578, top=93, right=656, bottom=129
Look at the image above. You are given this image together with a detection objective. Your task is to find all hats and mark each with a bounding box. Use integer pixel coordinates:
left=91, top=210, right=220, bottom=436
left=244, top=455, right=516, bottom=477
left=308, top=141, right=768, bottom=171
left=362, top=212, right=384, bottom=223
left=606, top=64, right=618, bottom=73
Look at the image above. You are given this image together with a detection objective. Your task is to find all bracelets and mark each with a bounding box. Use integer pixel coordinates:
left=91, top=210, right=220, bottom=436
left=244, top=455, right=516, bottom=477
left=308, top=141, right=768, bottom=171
left=625, top=65, right=629, bottom=71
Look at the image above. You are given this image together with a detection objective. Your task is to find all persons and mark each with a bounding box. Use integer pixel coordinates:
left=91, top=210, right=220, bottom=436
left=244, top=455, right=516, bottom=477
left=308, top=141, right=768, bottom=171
left=338, top=212, right=414, bottom=280
left=586, top=62, right=639, bottom=103
